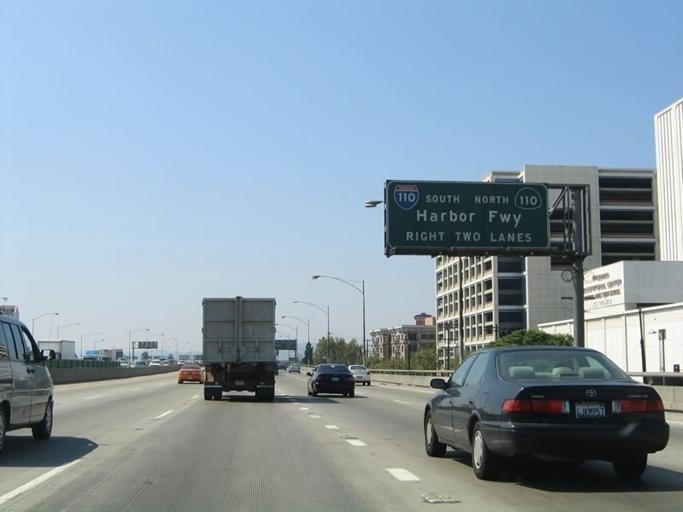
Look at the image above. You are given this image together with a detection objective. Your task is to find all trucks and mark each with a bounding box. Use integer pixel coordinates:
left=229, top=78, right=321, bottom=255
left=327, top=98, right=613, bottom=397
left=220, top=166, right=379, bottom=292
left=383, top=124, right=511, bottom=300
left=201, top=297, right=278, bottom=402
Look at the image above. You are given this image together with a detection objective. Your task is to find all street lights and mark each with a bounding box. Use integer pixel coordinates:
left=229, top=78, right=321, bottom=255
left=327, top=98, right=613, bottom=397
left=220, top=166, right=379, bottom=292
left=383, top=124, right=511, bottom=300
left=365, top=200, right=383, bottom=208
left=32, top=313, right=105, bottom=360
left=0, top=297, right=8, bottom=300
left=274, top=315, right=310, bottom=364
left=128, top=328, right=191, bottom=367
left=294, top=300, right=331, bottom=366
left=312, top=274, right=367, bottom=367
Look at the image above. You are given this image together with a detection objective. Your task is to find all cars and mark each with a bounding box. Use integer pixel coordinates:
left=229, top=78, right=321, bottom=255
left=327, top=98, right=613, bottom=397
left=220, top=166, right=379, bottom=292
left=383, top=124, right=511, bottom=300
left=286, top=364, right=301, bottom=374
left=421, top=344, right=670, bottom=483
left=120, top=356, right=184, bottom=367
left=347, top=364, right=371, bottom=387
left=177, top=365, right=205, bottom=384
left=306, top=364, right=356, bottom=399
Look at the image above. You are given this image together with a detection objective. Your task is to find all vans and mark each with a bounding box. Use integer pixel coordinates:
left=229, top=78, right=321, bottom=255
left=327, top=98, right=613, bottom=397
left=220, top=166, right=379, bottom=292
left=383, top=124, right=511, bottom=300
left=0, top=314, right=54, bottom=445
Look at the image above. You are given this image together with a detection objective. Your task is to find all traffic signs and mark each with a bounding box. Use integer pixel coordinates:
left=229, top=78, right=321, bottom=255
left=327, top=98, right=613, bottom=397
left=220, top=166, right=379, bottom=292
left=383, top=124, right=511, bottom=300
left=383, top=179, right=551, bottom=257
